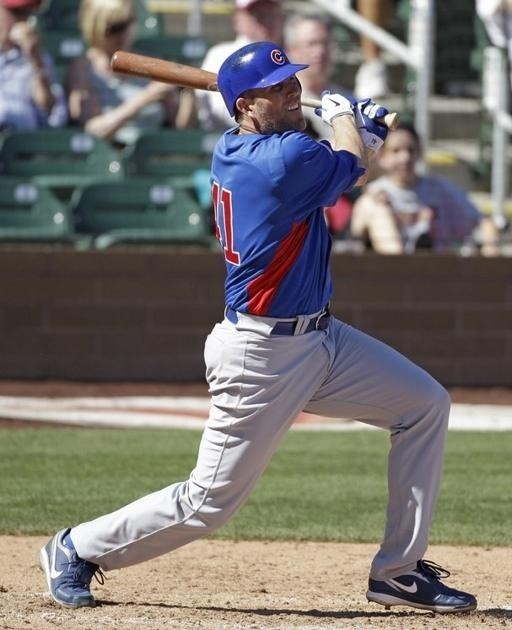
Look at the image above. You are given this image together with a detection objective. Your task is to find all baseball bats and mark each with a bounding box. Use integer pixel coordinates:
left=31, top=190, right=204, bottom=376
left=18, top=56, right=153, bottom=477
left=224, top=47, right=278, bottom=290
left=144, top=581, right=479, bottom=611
left=111, top=50, right=401, bottom=133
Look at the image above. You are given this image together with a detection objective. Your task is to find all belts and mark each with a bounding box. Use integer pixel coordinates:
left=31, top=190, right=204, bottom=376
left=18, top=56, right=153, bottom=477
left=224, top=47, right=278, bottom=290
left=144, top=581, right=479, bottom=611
left=224, top=300, right=332, bottom=336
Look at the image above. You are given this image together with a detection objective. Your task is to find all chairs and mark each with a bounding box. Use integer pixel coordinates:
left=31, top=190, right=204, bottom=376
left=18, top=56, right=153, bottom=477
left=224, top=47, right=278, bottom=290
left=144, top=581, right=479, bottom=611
left=0, top=180, right=74, bottom=243
left=95, top=227, right=220, bottom=250
left=0, top=130, right=128, bottom=175
left=123, top=131, right=225, bottom=177
left=39, top=1, right=213, bottom=80
left=69, top=176, right=210, bottom=235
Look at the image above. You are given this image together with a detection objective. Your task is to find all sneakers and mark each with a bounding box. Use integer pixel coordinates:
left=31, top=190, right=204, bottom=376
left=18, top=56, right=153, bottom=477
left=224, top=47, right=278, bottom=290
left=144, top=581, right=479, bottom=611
left=37, top=525, right=112, bottom=610
left=365, top=557, right=478, bottom=616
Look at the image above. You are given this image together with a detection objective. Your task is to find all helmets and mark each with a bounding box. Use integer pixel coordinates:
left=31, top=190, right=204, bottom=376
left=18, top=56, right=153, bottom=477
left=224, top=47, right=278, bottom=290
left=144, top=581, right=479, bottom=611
left=217, top=41, right=311, bottom=119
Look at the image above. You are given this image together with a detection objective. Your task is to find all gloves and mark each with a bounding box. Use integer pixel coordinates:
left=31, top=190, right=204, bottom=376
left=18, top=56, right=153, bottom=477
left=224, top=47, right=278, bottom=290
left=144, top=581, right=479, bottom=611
left=314, top=88, right=356, bottom=127
left=350, top=97, right=395, bottom=156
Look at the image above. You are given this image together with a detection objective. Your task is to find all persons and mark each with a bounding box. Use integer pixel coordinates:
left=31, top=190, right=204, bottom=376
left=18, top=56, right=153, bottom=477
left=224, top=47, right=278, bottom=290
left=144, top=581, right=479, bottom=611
left=0, top=0, right=69, bottom=131
left=42, top=42, right=477, bottom=614
left=64, top=0, right=197, bottom=151
left=198, top=0, right=486, bottom=253
